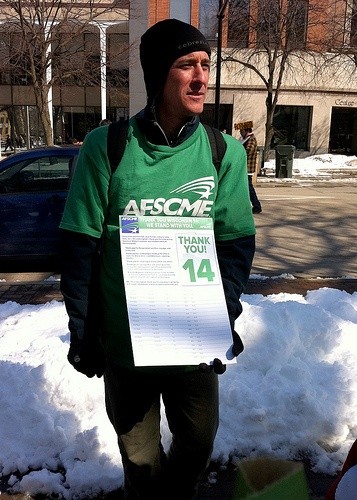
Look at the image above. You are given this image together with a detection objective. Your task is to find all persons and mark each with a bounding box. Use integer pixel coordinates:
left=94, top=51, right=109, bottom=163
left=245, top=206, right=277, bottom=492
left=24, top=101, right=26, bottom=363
left=2, top=135, right=13, bottom=152
left=100, top=119, right=111, bottom=126
left=57, top=135, right=62, bottom=144
left=10, top=135, right=24, bottom=148
left=59, top=18, right=256, bottom=500
left=240, top=128, right=262, bottom=214
left=221, top=128, right=226, bottom=133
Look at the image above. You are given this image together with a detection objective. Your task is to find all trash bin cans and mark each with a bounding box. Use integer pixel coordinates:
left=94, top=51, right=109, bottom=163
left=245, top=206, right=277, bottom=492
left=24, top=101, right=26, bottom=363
left=256, top=149, right=265, bottom=176
left=274, top=145, right=295, bottom=178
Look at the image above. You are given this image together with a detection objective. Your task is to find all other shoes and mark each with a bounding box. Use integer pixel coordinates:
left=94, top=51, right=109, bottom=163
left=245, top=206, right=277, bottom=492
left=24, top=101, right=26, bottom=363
left=252, top=210, right=261, bottom=214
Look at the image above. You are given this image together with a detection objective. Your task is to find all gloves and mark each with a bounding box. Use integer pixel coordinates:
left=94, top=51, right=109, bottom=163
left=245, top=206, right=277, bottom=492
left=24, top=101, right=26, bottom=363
left=199, top=316, right=243, bottom=377
left=68, top=338, right=105, bottom=378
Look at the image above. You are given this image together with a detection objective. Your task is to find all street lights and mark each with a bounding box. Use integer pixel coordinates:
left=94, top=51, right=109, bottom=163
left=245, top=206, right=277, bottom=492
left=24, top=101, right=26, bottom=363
left=80, top=19, right=129, bottom=120
left=32, top=19, right=61, bottom=145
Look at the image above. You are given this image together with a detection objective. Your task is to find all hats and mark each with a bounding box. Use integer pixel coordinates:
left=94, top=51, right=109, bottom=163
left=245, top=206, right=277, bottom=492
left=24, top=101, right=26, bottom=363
left=140, top=19, right=211, bottom=97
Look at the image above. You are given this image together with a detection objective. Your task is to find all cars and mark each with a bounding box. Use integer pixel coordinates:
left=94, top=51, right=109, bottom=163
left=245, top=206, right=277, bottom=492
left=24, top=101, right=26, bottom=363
left=0, top=144, right=83, bottom=271
